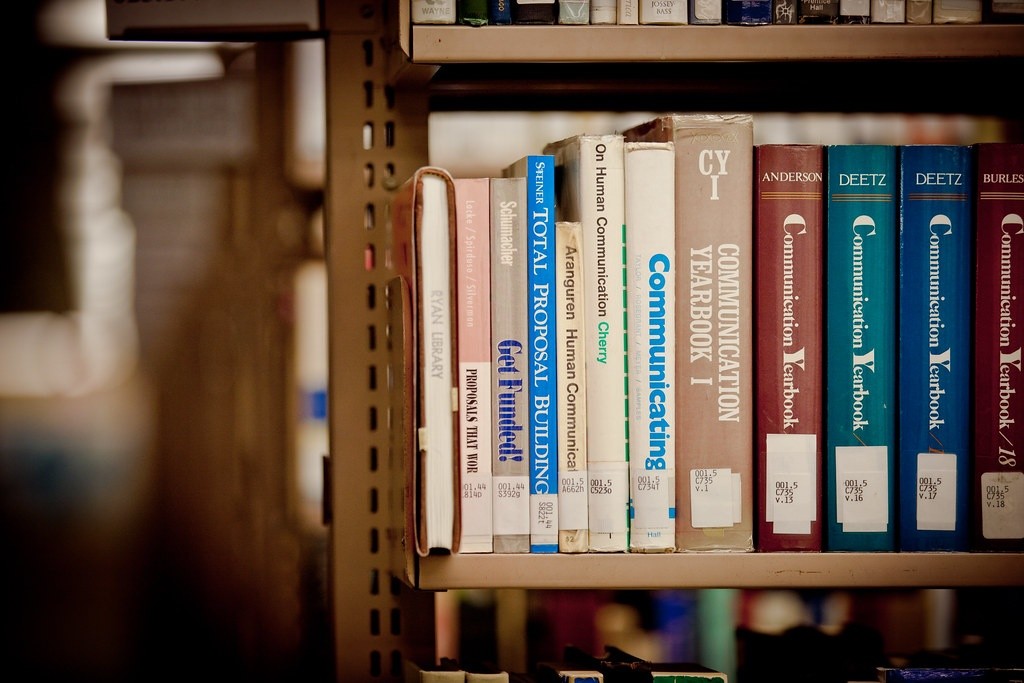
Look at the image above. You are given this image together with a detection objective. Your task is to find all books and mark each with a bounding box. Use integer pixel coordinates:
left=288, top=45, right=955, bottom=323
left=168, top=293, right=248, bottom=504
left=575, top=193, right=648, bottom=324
left=407, top=587, right=1024, bottom=683
left=392, top=114, right=1024, bottom=554
left=413, top=0, right=1024, bottom=26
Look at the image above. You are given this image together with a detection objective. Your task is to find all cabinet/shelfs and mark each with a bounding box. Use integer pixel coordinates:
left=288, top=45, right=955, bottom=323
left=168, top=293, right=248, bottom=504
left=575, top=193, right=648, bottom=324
left=81, top=0, right=1024, bottom=682
